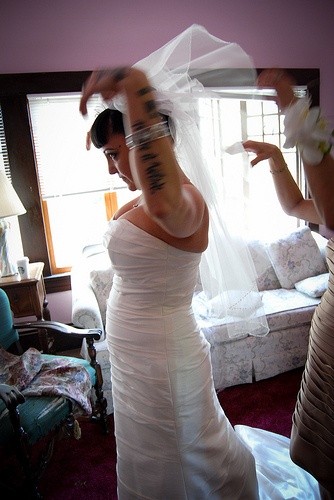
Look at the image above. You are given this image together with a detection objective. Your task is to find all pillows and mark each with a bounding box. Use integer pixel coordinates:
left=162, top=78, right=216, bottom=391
left=294, top=271, right=332, bottom=298
left=87, top=263, right=115, bottom=324
left=244, top=227, right=280, bottom=291
left=206, top=288, right=264, bottom=316
left=266, top=226, right=329, bottom=290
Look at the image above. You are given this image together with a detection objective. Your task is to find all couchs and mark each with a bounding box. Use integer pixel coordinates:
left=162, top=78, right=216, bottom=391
left=68, top=225, right=330, bottom=416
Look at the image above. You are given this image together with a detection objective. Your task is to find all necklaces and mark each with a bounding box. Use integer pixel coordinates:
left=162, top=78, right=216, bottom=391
left=132, top=196, right=143, bottom=208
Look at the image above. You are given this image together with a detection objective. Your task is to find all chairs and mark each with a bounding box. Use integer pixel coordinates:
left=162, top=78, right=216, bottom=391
left=0, top=288, right=110, bottom=500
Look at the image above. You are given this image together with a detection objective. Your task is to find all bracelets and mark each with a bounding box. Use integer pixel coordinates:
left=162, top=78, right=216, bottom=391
left=124, top=121, right=171, bottom=149
left=271, top=164, right=288, bottom=175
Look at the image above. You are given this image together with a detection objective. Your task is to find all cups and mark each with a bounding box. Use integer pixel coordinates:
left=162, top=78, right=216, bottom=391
left=16, top=257, right=30, bottom=280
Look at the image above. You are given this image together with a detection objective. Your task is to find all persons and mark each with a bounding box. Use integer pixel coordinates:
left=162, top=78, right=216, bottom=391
left=80, top=65, right=259, bottom=500
left=225, top=73, right=334, bottom=495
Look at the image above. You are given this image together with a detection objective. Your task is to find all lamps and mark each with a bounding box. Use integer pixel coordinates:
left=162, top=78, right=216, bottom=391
left=0, top=168, right=28, bottom=278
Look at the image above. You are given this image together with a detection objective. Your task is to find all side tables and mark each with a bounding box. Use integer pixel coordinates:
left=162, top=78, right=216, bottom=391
left=0, top=262, right=47, bottom=321
